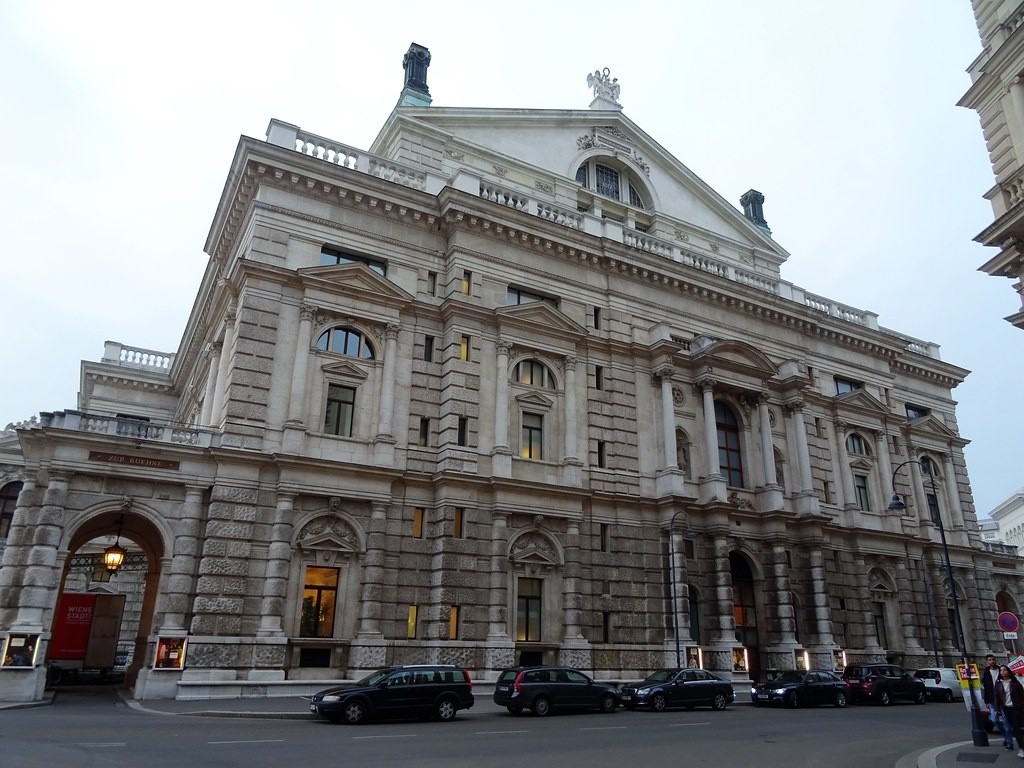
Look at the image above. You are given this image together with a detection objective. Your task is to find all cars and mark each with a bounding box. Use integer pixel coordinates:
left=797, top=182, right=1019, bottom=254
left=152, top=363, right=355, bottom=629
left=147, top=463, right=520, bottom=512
left=750, top=667, right=854, bottom=710
left=913, top=667, right=964, bottom=703
left=618, top=667, right=737, bottom=713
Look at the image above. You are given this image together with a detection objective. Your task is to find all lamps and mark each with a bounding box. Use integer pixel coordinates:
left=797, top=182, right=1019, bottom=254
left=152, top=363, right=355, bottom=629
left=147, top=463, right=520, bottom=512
left=104, top=515, right=128, bottom=576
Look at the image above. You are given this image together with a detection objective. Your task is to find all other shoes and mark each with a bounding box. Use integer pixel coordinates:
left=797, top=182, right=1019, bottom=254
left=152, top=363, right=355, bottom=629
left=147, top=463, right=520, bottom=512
left=1016, top=749, right=1024, bottom=759
left=1003, top=740, right=1007, bottom=746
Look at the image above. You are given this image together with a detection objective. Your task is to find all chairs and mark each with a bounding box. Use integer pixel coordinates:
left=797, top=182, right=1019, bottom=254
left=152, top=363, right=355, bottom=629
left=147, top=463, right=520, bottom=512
left=855, top=669, right=859, bottom=676
left=542, top=672, right=551, bottom=682
left=685, top=672, right=697, bottom=681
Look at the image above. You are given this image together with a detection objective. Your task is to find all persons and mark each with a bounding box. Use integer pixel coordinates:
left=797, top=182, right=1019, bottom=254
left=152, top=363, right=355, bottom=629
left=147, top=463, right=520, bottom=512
left=994, top=665, right=1024, bottom=758
left=981, top=654, right=1014, bottom=750
left=10, top=645, right=34, bottom=666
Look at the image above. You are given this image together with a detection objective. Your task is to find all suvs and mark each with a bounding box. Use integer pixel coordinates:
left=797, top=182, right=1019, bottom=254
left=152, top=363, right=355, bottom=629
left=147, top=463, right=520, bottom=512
left=839, top=661, right=928, bottom=706
left=309, top=664, right=474, bottom=726
left=493, top=663, right=622, bottom=717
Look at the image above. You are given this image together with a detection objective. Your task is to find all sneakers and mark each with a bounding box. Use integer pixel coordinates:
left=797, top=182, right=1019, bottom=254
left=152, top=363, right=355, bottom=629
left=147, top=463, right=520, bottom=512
left=1005, top=745, right=1014, bottom=751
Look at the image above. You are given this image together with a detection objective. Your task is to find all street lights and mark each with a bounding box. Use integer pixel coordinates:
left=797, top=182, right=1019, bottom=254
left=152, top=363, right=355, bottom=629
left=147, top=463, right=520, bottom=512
left=920, top=548, right=948, bottom=668
left=671, top=510, right=696, bottom=669
left=887, top=460, right=991, bottom=747
